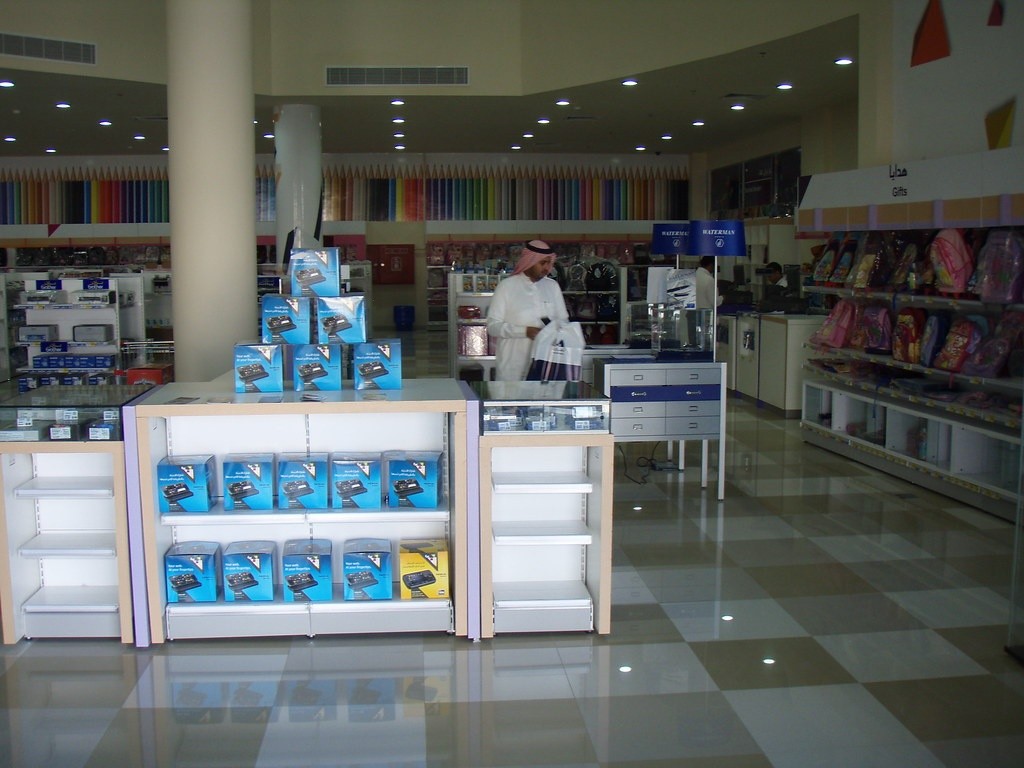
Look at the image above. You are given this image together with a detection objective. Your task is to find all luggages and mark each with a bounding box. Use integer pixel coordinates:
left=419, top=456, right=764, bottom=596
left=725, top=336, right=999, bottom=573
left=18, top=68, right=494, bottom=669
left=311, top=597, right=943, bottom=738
left=427, top=241, right=677, bottom=324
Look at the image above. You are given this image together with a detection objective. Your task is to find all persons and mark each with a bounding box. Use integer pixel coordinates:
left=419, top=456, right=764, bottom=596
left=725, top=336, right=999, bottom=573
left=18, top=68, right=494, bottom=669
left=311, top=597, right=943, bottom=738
left=486, top=239, right=569, bottom=382
left=682, top=256, right=724, bottom=351
left=766, top=262, right=788, bottom=288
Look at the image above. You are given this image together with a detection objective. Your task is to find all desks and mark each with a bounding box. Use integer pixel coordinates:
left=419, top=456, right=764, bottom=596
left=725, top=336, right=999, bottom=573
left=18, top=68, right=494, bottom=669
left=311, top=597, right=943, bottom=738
left=594, top=360, right=732, bottom=499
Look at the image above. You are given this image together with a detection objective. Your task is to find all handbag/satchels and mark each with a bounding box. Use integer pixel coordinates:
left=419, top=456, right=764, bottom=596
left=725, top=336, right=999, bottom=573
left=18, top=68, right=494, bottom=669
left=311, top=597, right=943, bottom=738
left=524, top=319, right=585, bottom=382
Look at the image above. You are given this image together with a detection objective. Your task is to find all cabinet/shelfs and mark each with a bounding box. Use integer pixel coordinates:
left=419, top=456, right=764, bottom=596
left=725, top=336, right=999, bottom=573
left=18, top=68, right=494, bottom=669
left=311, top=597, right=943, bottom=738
left=0, top=438, right=134, bottom=644
left=480, top=399, right=617, bottom=637
left=802, top=285, right=1024, bottom=527
left=0, top=271, right=145, bottom=391
left=426, top=242, right=653, bottom=380
left=124, top=381, right=478, bottom=642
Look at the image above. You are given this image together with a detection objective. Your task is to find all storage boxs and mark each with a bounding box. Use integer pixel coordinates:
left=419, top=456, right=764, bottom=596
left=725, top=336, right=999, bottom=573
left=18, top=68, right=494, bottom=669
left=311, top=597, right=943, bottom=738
left=489, top=407, right=597, bottom=431
left=235, top=247, right=405, bottom=392
left=26, top=279, right=115, bottom=306
left=283, top=539, right=333, bottom=602
left=400, top=538, right=450, bottom=599
left=343, top=539, right=392, bottom=603
left=165, top=541, right=221, bottom=603
left=332, top=451, right=384, bottom=510
left=387, top=452, right=442, bottom=508
left=33, top=353, right=114, bottom=369
left=222, top=541, right=279, bottom=601
left=19, top=325, right=58, bottom=342
left=223, top=452, right=275, bottom=509
left=460, top=326, right=498, bottom=356
left=17, top=373, right=113, bottom=394
left=0, top=410, right=120, bottom=442
left=73, top=324, right=112, bottom=343
left=279, top=453, right=327, bottom=508
left=158, top=453, right=216, bottom=515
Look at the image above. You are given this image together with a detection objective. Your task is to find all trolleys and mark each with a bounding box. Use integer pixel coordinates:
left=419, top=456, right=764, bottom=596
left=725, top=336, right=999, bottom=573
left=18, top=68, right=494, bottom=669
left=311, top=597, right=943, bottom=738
left=114, top=340, right=174, bottom=389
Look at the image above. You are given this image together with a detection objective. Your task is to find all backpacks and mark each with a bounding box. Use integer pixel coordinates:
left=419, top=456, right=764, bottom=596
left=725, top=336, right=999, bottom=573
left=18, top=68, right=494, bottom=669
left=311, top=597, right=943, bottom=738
left=801, top=225, right=1023, bottom=423
left=15, top=244, right=171, bottom=277
